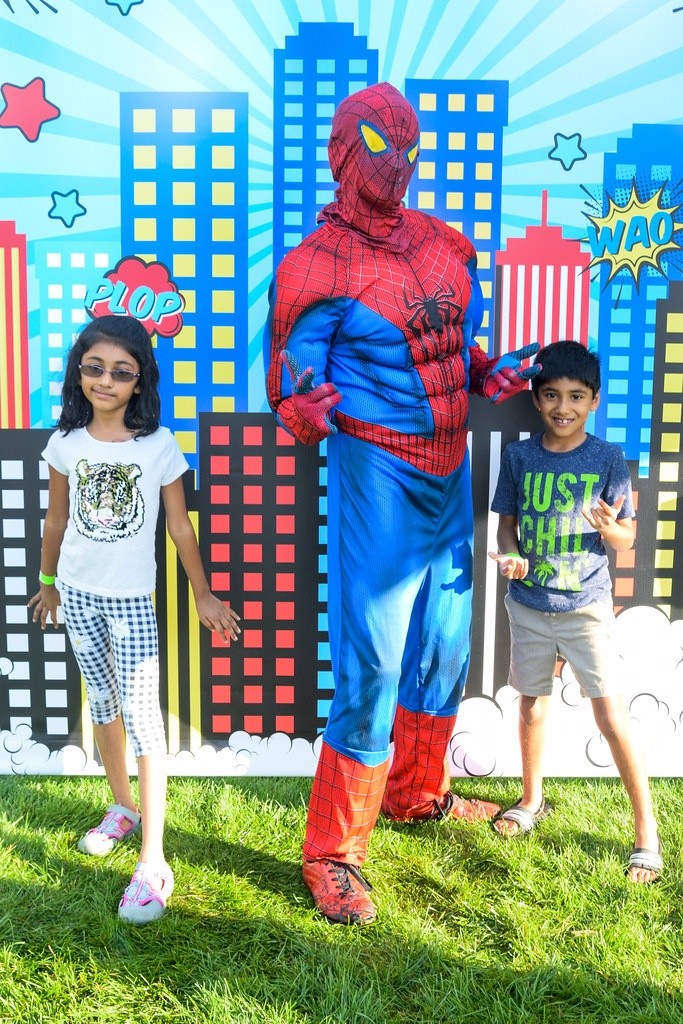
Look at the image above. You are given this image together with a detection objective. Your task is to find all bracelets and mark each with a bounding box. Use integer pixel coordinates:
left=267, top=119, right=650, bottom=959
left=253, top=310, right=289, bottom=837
left=505, top=553, right=520, bottom=558
left=38, top=570, right=55, bottom=585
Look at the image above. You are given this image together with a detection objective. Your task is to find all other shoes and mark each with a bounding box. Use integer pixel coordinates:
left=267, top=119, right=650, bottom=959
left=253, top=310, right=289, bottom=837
left=78, top=804, right=144, bottom=856
left=117, top=861, right=174, bottom=923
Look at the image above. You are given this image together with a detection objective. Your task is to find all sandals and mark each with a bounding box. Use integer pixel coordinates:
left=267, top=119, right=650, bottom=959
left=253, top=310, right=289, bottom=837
left=624, top=837, right=664, bottom=886
left=493, top=792, right=553, bottom=839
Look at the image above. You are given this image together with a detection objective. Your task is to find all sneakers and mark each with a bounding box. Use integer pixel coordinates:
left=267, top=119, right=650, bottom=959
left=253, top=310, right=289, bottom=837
left=302, top=858, right=377, bottom=925
left=421, top=791, right=502, bottom=824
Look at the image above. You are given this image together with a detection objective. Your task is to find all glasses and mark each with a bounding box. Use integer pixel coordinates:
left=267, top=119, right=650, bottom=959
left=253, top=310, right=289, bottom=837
left=77, top=356, right=141, bottom=383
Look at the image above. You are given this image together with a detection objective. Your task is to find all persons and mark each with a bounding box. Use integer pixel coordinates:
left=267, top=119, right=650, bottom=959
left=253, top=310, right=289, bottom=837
left=27, top=315, right=240, bottom=922
left=488, top=340, right=665, bottom=886
left=263, top=83, right=542, bottom=924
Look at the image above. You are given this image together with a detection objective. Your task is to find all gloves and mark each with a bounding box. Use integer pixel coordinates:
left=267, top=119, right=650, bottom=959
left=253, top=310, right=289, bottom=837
left=280, top=350, right=343, bottom=436
left=485, top=342, right=542, bottom=406
